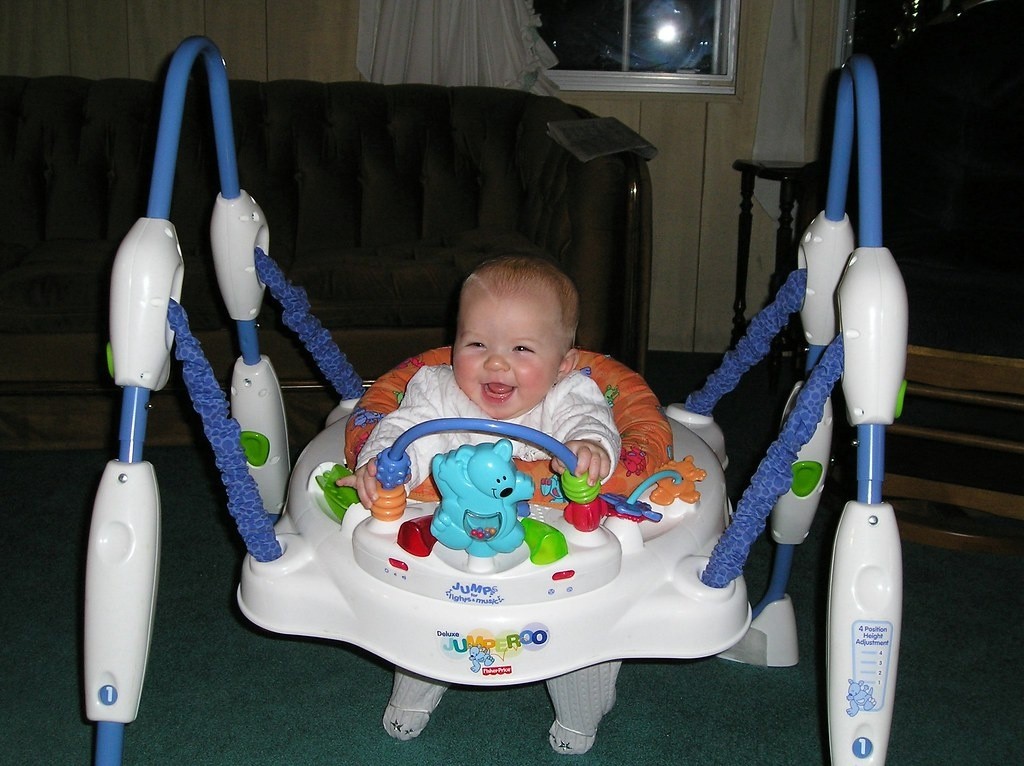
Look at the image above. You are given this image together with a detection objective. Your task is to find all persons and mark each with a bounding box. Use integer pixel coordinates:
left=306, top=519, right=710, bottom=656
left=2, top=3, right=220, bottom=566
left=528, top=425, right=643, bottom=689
left=336, top=254, right=622, bottom=755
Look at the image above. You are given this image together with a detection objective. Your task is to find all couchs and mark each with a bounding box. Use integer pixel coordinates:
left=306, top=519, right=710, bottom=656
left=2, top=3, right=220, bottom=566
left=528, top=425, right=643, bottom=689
left=0, top=73, right=654, bottom=452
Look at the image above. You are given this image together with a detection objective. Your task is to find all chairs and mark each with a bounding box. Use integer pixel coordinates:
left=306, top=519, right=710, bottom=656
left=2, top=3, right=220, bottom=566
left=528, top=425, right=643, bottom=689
left=791, top=0, right=1023, bottom=554
